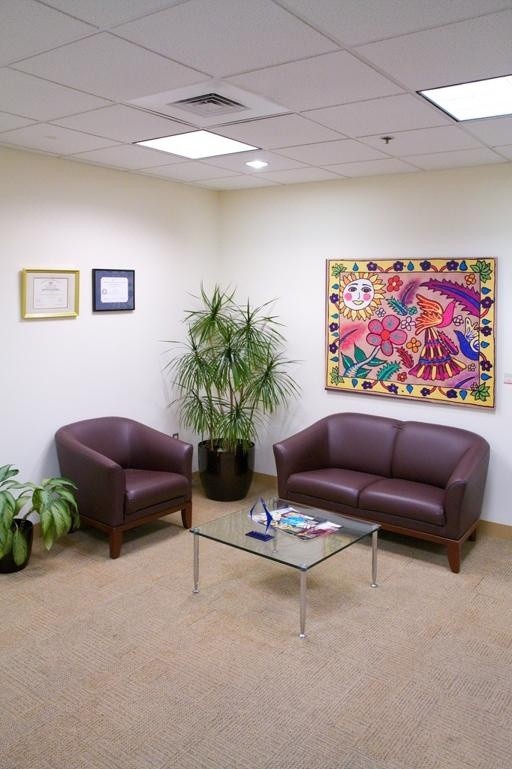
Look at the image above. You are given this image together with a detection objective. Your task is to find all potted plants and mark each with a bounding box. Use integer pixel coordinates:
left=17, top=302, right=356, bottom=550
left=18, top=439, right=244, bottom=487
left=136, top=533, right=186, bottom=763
left=159, top=280, right=303, bottom=503
left=0, top=462, right=80, bottom=575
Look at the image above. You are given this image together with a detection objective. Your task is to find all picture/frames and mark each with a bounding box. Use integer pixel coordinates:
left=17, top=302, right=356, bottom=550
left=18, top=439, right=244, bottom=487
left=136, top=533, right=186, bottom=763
left=322, top=256, right=498, bottom=412
left=19, top=267, right=81, bottom=321
left=90, top=267, right=136, bottom=313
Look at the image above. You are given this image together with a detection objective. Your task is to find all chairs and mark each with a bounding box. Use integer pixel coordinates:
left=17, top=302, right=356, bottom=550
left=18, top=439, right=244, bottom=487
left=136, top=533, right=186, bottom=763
left=54, top=415, right=194, bottom=558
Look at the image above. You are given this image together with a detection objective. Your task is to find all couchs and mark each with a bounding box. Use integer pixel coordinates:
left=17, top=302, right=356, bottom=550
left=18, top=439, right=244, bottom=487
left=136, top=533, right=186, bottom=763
left=271, top=412, right=491, bottom=574
